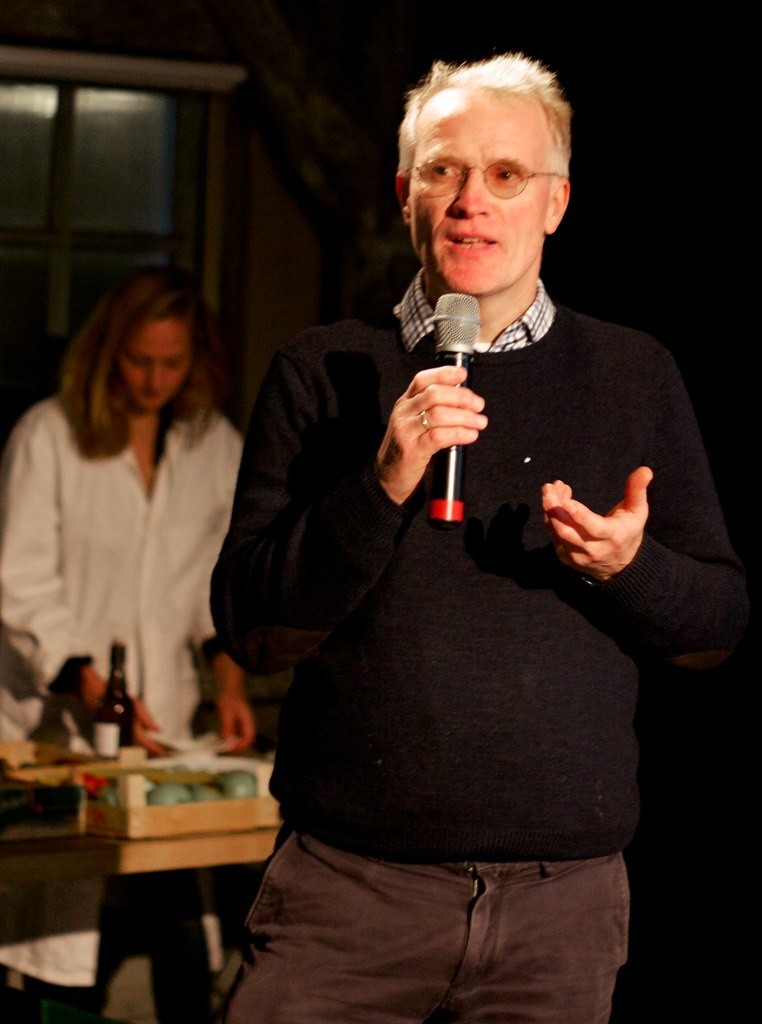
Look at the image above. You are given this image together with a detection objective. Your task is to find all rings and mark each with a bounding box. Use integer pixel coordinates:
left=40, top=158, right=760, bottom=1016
left=420, top=410, right=432, bottom=432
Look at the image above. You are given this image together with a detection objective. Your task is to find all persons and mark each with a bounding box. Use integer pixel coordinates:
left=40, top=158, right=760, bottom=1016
left=203, top=47, right=759, bottom=1024
left=0, top=257, right=261, bottom=1024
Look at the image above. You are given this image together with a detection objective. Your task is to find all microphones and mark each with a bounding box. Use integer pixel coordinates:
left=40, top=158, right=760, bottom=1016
left=429, top=293, right=483, bottom=530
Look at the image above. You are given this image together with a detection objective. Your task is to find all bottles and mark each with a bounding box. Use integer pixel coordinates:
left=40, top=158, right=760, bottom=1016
left=92, top=641, right=136, bottom=760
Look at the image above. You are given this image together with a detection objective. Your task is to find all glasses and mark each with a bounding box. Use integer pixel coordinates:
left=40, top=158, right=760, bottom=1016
left=412, top=155, right=569, bottom=201
